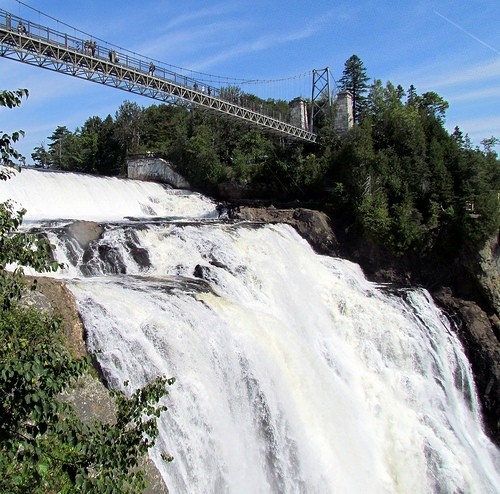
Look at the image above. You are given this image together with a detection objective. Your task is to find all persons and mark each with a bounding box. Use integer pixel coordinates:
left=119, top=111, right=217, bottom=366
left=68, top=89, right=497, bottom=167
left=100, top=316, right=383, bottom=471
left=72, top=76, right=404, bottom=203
left=79, top=38, right=263, bottom=119
